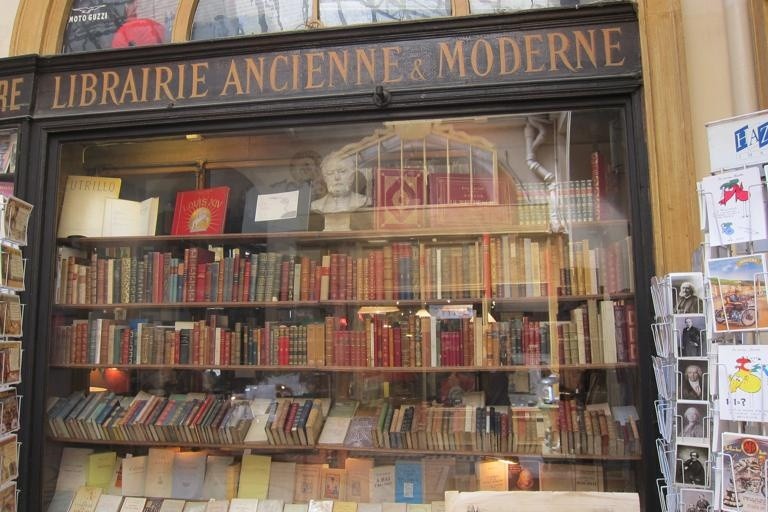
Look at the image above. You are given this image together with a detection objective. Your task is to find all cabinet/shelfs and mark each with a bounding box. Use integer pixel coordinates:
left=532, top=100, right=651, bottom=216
left=41, top=217, right=643, bottom=511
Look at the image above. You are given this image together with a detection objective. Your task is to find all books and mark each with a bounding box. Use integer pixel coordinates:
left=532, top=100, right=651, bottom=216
left=58, top=175, right=311, bottom=238
left=1, top=194, right=37, bottom=512
left=54, top=299, right=637, bottom=366
left=695, top=164, right=768, bottom=511
left=374, top=152, right=618, bottom=232
left=55, top=232, right=632, bottom=304
left=47, top=448, right=642, bottom=512
left=46, top=389, right=642, bottom=459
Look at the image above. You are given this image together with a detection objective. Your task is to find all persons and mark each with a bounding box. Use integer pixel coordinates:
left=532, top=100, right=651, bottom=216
left=675, top=280, right=713, bottom=511
left=307, top=151, right=373, bottom=213
left=445, top=373, right=466, bottom=408
